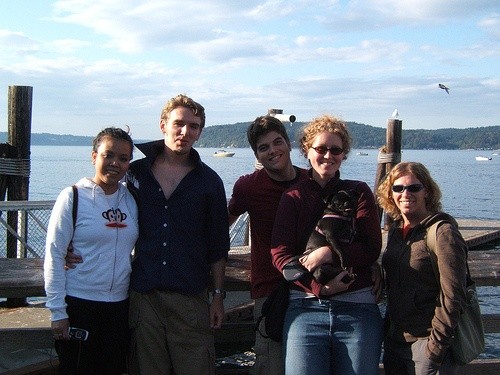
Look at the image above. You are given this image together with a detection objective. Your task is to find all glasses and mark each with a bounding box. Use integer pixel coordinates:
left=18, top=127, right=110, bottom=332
left=310, top=146, right=345, bottom=155
left=392, top=183, right=424, bottom=193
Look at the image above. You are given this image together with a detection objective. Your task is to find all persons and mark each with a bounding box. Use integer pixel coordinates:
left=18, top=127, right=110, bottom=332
left=228, top=116, right=385, bottom=374
left=64, top=94, right=230, bottom=375
left=270, top=114, right=383, bottom=375
left=43, top=94, right=138, bottom=375
left=375, top=161, right=469, bottom=375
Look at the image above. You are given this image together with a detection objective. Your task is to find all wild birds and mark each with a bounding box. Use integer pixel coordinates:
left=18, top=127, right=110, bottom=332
left=439, top=83, right=450, bottom=95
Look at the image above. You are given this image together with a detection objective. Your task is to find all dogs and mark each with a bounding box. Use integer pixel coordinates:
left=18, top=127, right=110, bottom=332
left=302, top=190, right=361, bottom=286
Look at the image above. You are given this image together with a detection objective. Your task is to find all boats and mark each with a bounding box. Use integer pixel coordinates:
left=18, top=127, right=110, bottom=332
left=209, top=150, right=235, bottom=157
left=356, top=152, right=368, bottom=156
left=474, top=156, right=491, bottom=160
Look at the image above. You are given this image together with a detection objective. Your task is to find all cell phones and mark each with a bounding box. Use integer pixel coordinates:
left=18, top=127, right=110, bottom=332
left=68, top=327, right=88, bottom=340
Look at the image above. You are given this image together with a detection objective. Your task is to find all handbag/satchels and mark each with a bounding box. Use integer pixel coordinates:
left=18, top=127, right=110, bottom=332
left=427, top=220, right=485, bottom=366
left=254, top=278, right=290, bottom=343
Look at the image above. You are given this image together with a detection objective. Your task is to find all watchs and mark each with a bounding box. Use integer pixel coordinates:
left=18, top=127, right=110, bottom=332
left=212, top=288, right=226, bottom=299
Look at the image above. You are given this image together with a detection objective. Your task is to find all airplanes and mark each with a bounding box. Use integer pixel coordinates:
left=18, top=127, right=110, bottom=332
left=438, top=84, right=450, bottom=95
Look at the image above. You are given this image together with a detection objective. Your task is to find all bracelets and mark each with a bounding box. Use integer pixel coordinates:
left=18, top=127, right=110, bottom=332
left=323, top=286, right=327, bottom=296
